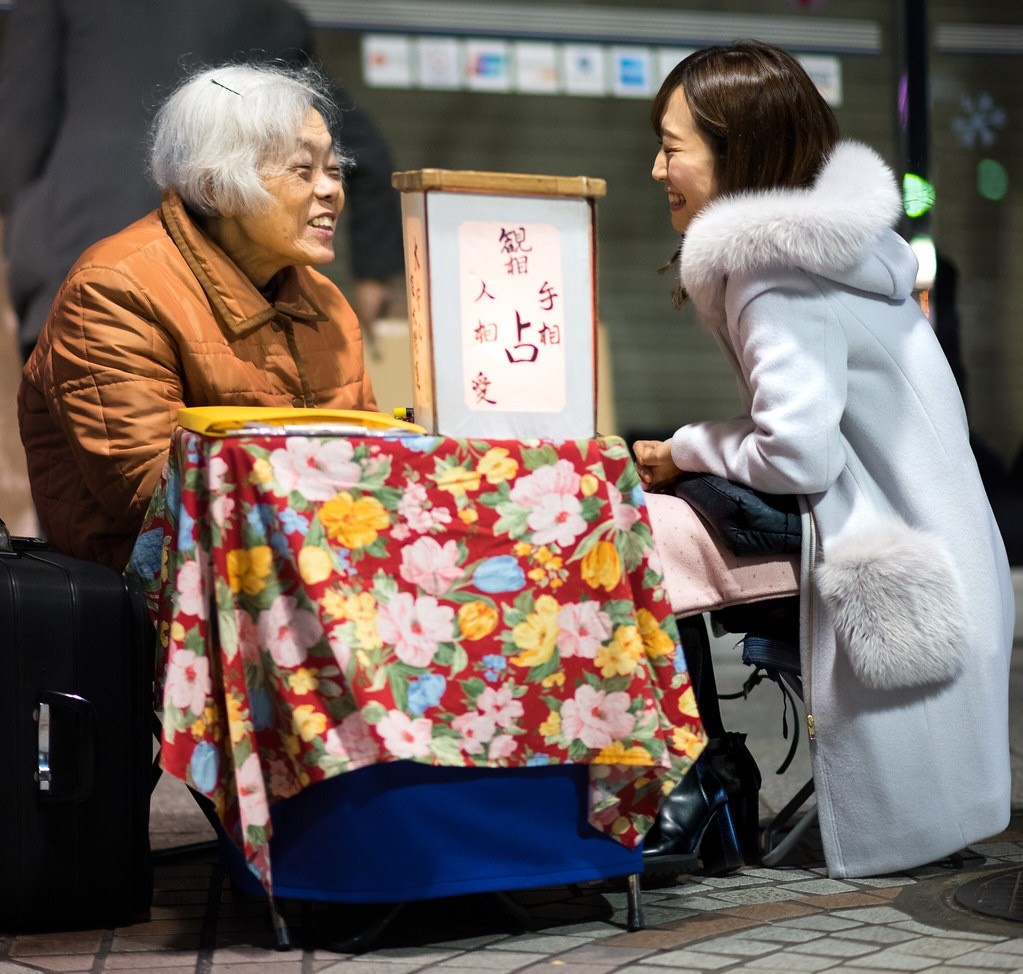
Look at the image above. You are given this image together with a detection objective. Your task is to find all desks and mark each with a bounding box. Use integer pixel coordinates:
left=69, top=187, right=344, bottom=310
left=163, top=424, right=715, bottom=949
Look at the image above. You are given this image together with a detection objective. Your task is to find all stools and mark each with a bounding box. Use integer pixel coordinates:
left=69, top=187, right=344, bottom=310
left=139, top=705, right=229, bottom=860
left=708, top=603, right=820, bottom=865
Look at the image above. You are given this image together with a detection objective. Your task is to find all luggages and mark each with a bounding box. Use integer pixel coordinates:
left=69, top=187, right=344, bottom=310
left=0, top=515, right=161, bottom=937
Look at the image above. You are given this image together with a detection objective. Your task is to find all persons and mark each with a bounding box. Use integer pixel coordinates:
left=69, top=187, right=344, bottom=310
left=0, top=0, right=373, bottom=367
left=332, top=84, right=402, bottom=323
left=15, top=64, right=383, bottom=577
left=624, top=36, right=1014, bottom=882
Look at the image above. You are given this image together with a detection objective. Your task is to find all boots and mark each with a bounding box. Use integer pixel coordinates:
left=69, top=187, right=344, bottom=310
left=639, top=730, right=762, bottom=877
